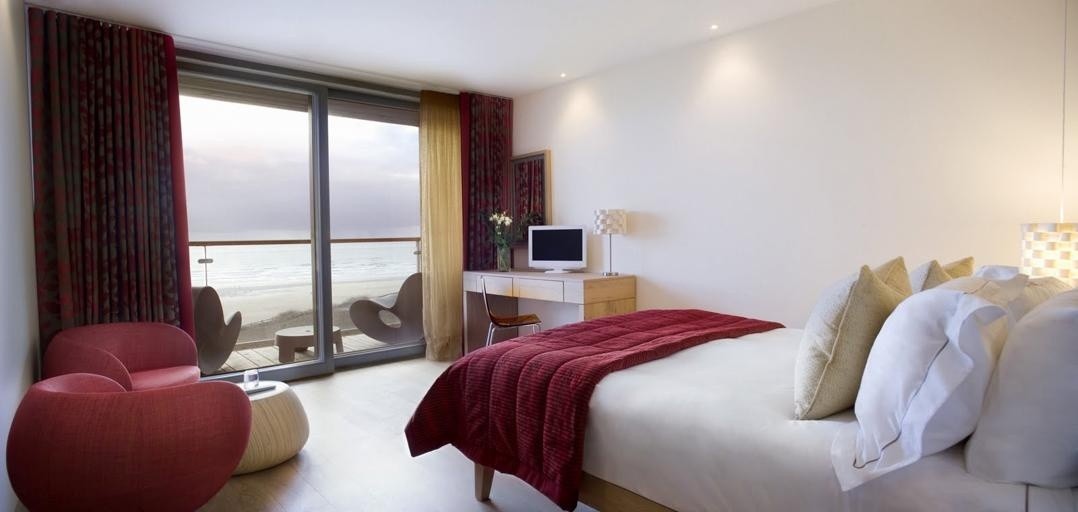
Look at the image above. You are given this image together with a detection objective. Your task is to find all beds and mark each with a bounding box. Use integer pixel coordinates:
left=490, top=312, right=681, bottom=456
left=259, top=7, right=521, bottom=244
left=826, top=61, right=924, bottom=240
left=398, top=306, right=1077, bottom=511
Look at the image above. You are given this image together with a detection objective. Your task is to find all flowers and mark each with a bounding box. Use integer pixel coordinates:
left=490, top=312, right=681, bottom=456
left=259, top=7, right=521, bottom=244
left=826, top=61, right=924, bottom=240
left=487, top=207, right=543, bottom=270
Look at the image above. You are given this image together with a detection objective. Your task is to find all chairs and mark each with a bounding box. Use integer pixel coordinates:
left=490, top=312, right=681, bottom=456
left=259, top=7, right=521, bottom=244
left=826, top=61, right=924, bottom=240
left=347, top=269, right=428, bottom=350
left=39, top=317, right=207, bottom=391
left=190, top=279, right=249, bottom=376
left=1, top=369, right=253, bottom=512
left=477, top=274, right=544, bottom=347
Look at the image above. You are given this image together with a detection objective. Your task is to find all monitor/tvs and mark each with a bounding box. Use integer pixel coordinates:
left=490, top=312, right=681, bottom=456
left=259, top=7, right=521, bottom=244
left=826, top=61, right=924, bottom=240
left=528, top=226, right=588, bottom=275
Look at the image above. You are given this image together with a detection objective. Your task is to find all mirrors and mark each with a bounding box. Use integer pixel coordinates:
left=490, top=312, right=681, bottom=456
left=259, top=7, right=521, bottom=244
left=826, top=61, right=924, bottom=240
left=510, top=148, right=552, bottom=251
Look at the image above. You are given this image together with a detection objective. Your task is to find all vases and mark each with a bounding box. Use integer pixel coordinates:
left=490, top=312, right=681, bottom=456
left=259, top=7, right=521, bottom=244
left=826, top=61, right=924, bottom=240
left=496, top=246, right=513, bottom=273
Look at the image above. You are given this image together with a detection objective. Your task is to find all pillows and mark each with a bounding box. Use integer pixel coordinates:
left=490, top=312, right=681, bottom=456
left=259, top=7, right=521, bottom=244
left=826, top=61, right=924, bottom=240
left=1011, top=267, right=1071, bottom=311
left=906, top=255, right=978, bottom=294
left=828, top=262, right=1021, bottom=493
left=789, top=252, right=917, bottom=427
left=962, top=286, right=1078, bottom=489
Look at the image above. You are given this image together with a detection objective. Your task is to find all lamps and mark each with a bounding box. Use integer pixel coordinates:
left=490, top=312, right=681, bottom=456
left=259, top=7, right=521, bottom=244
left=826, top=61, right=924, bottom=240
left=592, top=207, right=630, bottom=278
left=1019, top=0, right=1077, bottom=285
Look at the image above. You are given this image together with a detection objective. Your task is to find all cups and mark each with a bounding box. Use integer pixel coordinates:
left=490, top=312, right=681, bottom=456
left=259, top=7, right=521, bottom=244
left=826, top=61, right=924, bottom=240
left=243, top=369, right=259, bottom=390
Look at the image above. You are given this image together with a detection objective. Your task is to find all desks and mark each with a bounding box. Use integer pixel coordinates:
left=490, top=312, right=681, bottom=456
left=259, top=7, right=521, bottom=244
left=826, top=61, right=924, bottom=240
left=225, top=376, right=312, bottom=476
left=461, top=267, right=637, bottom=359
left=272, top=324, right=346, bottom=363
